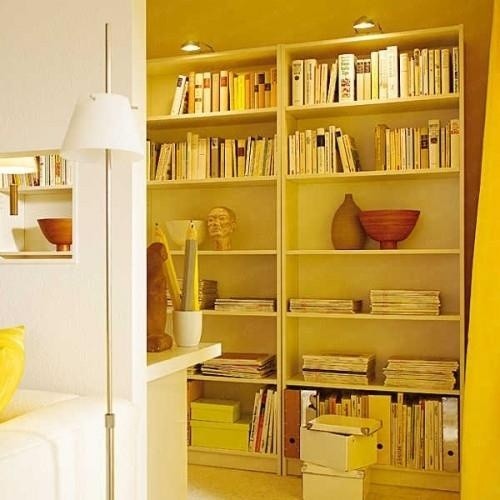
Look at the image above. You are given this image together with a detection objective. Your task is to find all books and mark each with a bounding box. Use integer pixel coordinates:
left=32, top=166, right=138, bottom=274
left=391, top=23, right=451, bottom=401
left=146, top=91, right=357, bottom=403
left=317, top=391, right=443, bottom=472
left=292, top=45, right=460, bottom=106
left=167, top=278, right=277, bottom=380
left=289, top=119, right=461, bottom=176
left=248, top=384, right=277, bottom=455
left=170, top=68, right=277, bottom=115
left=150, top=132, right=277, bottom=181
left=1, top=154, right=72, bottom=189
left=288, top=289, right=441, bottom=316
left=301, top=353, right=459, bottom=390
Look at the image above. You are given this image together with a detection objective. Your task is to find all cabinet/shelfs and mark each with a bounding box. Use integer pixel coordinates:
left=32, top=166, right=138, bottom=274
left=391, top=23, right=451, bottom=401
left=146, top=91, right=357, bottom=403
left=2, top=184, right=73, bottom=260
left=150, top=48, right=281, bottom=492
left=281, top=25, right=464, bottom=495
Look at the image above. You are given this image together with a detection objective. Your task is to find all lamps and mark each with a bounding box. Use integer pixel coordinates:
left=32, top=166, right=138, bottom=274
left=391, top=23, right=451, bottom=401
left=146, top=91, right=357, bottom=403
left=67, top=90, right=146, bottom=499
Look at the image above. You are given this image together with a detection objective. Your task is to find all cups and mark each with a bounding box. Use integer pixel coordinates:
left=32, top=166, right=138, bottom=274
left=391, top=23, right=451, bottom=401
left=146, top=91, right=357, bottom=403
left=172, top=310, right=203, bottom=347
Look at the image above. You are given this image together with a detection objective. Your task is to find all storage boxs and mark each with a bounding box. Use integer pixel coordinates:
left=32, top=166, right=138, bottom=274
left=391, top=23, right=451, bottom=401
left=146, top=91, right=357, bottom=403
left=300, top=464, right=372, bottom=500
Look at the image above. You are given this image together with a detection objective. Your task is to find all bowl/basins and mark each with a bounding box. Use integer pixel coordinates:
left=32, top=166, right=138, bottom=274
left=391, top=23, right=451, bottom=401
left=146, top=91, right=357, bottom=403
left=165, top=219, right=207, bottom=251
